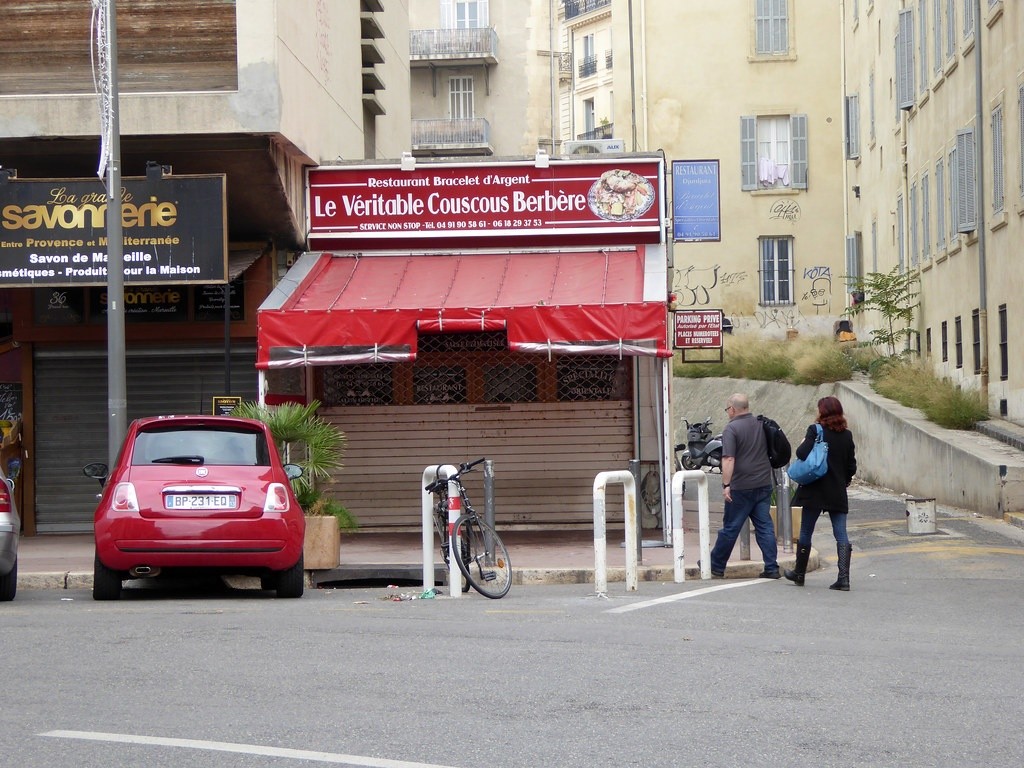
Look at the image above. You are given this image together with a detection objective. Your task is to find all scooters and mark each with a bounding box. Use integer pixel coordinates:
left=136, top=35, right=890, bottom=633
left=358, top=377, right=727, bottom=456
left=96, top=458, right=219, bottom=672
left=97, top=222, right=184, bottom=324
left=678, top=416, right=722, bottom=476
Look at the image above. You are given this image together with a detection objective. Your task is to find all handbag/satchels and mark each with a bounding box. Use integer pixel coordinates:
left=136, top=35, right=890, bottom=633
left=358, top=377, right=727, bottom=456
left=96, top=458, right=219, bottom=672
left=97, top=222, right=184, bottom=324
left=756, top=414, right=791, bottom=468
left=787, top=423, right=828, bottom=484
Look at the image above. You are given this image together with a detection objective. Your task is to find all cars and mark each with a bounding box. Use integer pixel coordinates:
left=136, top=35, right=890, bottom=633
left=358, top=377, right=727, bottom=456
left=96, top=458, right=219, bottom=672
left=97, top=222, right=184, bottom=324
left=82, top=414, right=307, bottom=600
left=0, top=457, right=20, bottom=602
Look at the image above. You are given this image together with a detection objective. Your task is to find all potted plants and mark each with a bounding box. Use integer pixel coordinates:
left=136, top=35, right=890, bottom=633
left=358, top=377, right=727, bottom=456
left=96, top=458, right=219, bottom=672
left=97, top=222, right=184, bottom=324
left=231, top=399, right=358, bottom=569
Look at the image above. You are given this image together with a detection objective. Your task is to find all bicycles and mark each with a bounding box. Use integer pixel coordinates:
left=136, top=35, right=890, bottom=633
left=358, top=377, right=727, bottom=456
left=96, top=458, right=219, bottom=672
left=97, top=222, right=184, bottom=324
left=423, top=457, right=513, bottom=601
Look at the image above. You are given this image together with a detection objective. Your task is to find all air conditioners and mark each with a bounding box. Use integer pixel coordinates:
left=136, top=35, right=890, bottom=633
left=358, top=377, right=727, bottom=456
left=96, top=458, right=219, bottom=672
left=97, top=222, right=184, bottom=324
left=560, top=139, right=624, bottom=159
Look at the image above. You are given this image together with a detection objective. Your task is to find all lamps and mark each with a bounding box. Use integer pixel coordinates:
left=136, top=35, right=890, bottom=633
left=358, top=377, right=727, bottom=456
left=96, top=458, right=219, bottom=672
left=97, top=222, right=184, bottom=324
left=535, top=149, right=549, bottom=168
left=145, top=161, right=172, bottom=181
left=401, top=152, right=415, bottom=170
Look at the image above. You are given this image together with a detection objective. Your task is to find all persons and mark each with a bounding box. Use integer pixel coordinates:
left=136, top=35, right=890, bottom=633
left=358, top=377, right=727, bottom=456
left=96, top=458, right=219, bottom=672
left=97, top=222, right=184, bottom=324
left=783, top=396, right=857, bottom=591
left=696, top=393, right=781, bottom=578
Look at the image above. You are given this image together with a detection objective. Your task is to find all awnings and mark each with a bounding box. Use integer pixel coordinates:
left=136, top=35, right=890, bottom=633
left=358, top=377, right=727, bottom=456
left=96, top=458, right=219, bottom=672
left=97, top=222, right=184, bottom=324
left=257, top=243, right=674, bottom=545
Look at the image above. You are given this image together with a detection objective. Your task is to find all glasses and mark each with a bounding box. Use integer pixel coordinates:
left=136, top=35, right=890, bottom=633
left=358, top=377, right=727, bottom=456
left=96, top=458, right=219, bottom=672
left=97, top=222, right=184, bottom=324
left=725, top=406, right=735, bottom=413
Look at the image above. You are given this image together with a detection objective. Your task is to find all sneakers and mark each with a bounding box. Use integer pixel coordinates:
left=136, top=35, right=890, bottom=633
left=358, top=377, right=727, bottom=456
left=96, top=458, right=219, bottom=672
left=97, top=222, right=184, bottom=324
left=697, top=560, right=725, bottom=578
left=758, top=570, right=780, bottom=578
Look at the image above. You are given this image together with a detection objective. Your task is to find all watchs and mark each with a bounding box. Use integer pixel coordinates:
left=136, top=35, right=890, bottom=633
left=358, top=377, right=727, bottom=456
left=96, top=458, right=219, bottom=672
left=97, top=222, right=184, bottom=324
left=721, top=483, right=730, bottom=489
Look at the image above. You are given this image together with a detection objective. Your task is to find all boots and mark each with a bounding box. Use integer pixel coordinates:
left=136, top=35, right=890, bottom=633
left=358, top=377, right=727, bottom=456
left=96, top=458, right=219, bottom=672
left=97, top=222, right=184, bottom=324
left=784, top=539, right=811, bottom=586
left=830, top=542, right=852, bottom=590
